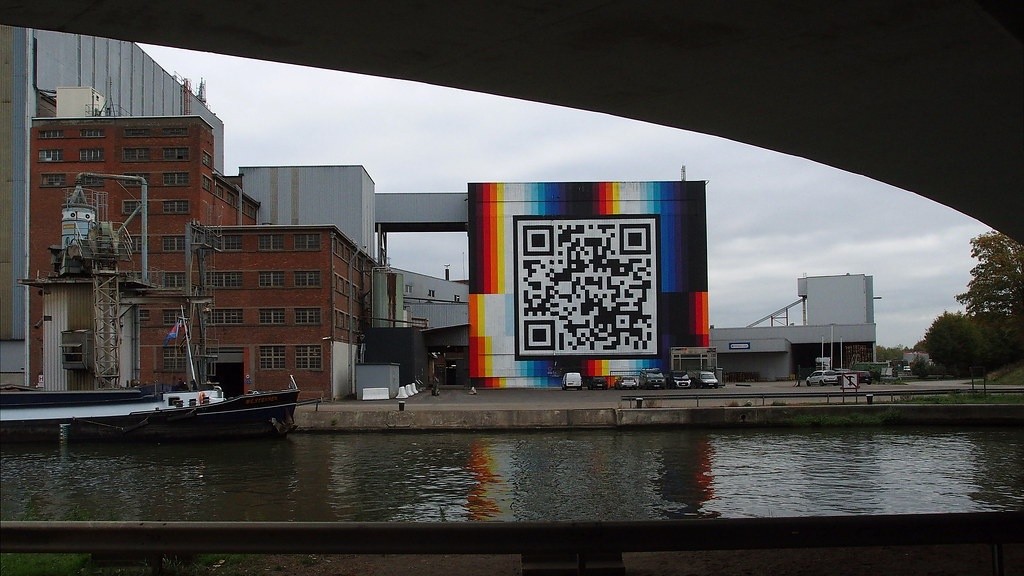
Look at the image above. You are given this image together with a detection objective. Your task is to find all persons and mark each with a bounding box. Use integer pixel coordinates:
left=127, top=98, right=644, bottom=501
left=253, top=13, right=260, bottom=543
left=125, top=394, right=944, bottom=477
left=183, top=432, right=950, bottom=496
left=431, top=376, right=440, bottom=396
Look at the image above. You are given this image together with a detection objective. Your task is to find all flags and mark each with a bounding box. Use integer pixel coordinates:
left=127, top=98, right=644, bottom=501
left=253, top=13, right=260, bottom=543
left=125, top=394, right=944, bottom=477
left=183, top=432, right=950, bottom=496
left=163, top=323, right=178, bottom=347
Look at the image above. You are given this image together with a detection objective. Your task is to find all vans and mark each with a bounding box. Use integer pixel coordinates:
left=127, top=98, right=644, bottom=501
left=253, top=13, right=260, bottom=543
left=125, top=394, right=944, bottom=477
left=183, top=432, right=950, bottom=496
left=562, top=372, right=582, bottom=390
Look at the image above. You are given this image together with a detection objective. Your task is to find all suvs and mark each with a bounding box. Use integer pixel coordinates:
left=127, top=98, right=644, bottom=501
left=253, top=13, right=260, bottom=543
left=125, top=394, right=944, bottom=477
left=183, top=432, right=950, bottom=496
left=640, top=370, right=667, bottom=390
left=588, top=376, right=607, bottom=389
left=695, top=371, right=719, bottom=389
left=855, top=371, right=872, bottom=385
left=670, top=371, right=691, bottom=389
left=806, top=370, right=839, bottom=386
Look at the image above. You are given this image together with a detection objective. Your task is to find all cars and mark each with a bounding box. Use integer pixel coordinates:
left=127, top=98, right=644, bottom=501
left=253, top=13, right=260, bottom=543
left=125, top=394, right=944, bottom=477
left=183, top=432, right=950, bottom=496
left=904, top=366, right=911, bottom=372
left=614, top=377, right=638, bottom=390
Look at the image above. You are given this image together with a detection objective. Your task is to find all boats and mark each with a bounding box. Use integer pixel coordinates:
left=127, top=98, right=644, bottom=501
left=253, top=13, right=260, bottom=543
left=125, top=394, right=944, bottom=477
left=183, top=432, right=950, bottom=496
left=0, top=375, right=301, bottom=437
